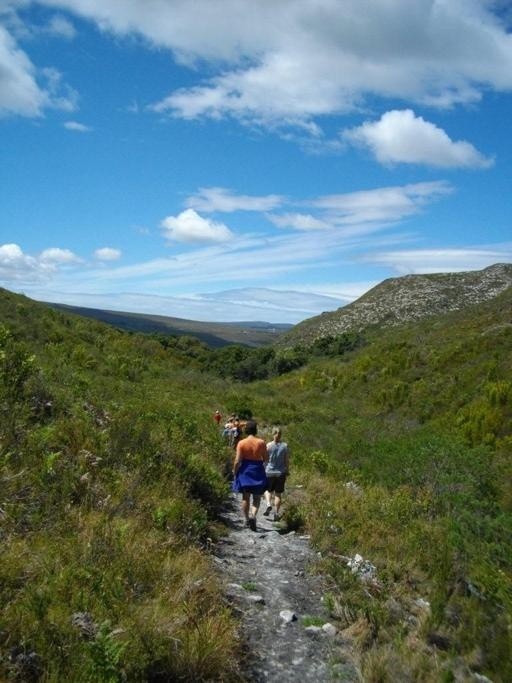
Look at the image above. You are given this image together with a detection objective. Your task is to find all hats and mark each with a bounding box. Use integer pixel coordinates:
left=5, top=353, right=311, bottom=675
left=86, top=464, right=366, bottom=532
left=271, top=427, right=280, bottom=435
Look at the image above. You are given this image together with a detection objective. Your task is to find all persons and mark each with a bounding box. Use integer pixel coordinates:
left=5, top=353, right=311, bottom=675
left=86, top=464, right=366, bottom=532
left=263, top=428, right=290, bottom=521
left=232, top=421, right=270, bottom=531
left=214, top=409, right=249, bottom=450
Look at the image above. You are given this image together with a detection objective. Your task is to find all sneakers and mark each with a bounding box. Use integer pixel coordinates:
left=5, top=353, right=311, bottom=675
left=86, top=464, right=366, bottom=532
left=264, top=505, right=271, bottom=516
left=249, top=517, right=256, bottom=531
